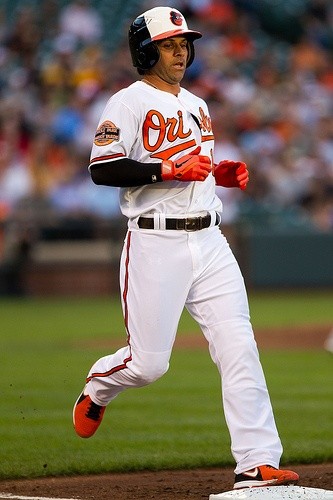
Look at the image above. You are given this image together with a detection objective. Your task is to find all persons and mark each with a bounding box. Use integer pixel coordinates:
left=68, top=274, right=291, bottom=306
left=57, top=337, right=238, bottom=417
left=73, top=7, right=299, bottom=489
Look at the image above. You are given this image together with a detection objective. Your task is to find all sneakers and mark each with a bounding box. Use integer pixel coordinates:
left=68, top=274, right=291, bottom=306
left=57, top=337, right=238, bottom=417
left=233, top=465, right=299, bottom=489
left=73, top=385, right=107, bottom=438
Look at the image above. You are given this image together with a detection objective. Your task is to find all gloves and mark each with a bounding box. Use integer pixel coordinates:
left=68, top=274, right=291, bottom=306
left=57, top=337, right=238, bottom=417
left=161, top=145, right=212, bottom=182
left=213, top=160, right=250, bottom=190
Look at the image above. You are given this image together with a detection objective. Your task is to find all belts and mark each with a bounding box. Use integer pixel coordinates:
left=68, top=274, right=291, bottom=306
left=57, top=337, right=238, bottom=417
left=138, top=214, right=219, bottom=232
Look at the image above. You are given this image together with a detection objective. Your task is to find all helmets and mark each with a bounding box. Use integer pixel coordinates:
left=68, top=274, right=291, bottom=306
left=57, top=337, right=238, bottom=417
left=129, top=7, right=202, bottom=74
